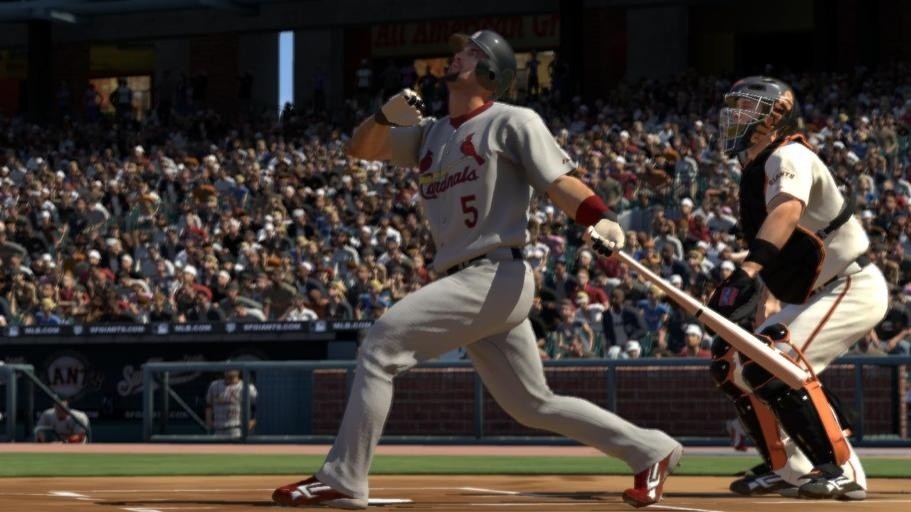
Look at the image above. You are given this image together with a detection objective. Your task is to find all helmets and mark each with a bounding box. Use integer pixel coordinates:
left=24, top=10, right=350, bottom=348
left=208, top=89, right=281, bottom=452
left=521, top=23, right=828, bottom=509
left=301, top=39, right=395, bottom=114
left=724, top=76, right=800, bottom=134
left=448, top=30, right=517, bottom=99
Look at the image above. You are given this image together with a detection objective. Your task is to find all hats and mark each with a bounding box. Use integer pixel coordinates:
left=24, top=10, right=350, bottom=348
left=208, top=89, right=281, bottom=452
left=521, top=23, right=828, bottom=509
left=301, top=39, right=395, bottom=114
left=1, top=144, right=399, bottom=309
left=545, top=96, right=872, bottom=356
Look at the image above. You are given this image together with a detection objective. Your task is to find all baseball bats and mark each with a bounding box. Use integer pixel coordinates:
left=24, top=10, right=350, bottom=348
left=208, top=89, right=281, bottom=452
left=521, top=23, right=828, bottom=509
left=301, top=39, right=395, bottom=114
left=582, top=224, right=808, bottom=391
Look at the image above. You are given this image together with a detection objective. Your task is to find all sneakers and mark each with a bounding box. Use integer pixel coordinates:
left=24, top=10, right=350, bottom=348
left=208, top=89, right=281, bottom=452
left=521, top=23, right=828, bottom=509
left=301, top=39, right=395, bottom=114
left=272, top=475, right=368, bottom=510
left=729, top=462, right=868, bottom=501
left=623, top=442, right=683, bottom=507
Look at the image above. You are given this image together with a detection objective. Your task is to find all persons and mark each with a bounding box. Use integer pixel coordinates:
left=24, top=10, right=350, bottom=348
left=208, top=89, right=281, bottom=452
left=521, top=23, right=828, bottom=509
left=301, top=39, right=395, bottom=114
left=513, top=68, right=910, bottom=360
left=205, top=369, right=258, bottom=435
left=271, top=30, right=684, bottom=509
left=34, top=400, right=92, bottom=442
left=707, top=75, right=890, bottom=499
left=0, top=65, right=438, bottom=330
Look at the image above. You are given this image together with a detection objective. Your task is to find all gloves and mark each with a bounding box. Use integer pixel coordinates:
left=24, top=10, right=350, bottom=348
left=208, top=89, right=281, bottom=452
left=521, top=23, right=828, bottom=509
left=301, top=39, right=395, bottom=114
left=375, top=88, right=425, bottom=129
